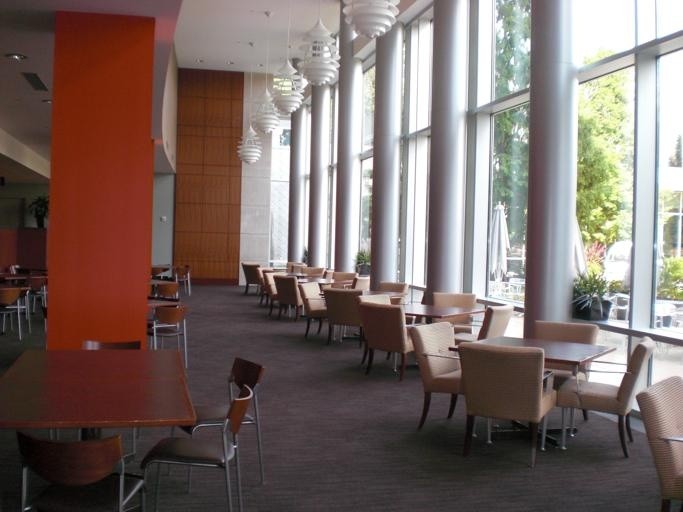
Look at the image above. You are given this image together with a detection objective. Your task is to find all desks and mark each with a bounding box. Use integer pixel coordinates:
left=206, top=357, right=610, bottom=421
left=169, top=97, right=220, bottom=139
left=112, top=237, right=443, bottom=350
left=0, top=350, right=198, bottom=440
left=448, top=335, right=616, bottom=449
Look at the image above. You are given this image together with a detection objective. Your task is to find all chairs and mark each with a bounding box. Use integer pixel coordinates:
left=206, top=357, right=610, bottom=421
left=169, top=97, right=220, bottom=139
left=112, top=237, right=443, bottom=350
left=167, top=356, right=267, bottom=494
left=531, top=320, right=599, bottom=421
left=409, top=321, right=464, bottom=430
left=241, top=262, right=514, bottom=382
left=143, top=382, right=253, bottom=510
left=636, top=376, right=683, bottom=512
left=458, top=342, right=558, bottom=469
left=1, top=258, right=194, bottom=370
left=556, top=335, right=657, bottom=458
left=15, top=433, right=146, bottom=512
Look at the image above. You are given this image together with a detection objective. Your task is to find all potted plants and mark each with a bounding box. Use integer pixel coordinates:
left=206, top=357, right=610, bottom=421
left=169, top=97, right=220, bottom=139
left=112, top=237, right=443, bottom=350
left=568, top=268, right=624, bottom=320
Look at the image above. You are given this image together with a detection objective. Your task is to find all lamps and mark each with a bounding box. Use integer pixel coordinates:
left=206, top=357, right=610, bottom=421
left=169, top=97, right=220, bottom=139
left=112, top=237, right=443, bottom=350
left=297, top=1, right=341, bottom=86
left=340, top=2, right=401, bottom=42
left=234, top=44, right=262, bottom=166
left=271, top=1, right=309, bottom=117
left=256, top=9, right=279, bottom=138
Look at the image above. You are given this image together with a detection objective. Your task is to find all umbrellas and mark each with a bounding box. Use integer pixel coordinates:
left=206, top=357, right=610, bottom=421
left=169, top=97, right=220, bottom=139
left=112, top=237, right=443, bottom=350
left=575, top=214, right=588, bottom=279
left=490, top=200, right=510, bottom=281
